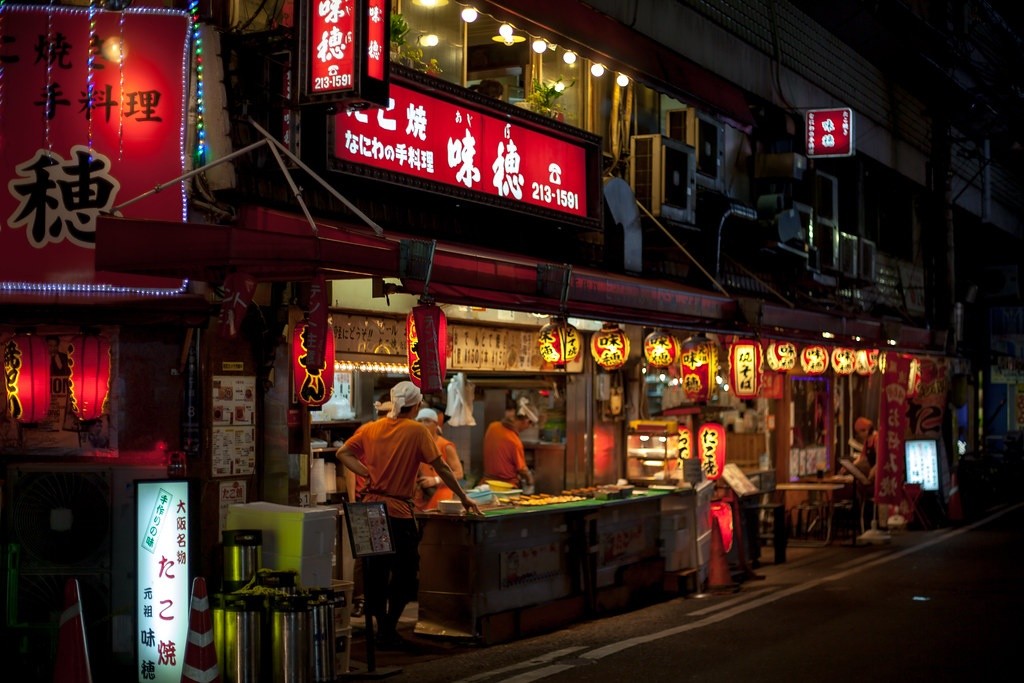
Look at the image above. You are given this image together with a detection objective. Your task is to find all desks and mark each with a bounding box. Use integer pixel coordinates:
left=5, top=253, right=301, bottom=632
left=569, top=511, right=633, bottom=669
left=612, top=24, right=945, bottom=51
left=776, top=483, right=846, bottom=550
left=417, top=489, right=670, bottom=632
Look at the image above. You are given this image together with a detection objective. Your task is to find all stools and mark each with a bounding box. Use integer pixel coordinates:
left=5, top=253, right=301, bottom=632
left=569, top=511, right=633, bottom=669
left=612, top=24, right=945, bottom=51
left=743, top=501, right=788, bottom=563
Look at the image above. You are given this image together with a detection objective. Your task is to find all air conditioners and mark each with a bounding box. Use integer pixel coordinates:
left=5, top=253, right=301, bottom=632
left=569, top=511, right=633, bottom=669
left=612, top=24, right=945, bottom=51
left=629, top=109, right=724, bottom=226
left=791, top=203, right=876, bottom=279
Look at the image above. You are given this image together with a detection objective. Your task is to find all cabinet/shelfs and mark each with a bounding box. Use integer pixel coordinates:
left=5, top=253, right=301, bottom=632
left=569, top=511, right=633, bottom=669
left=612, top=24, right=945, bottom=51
left=660, top=478, right=712, bottom=583
left=310, top=418, right=359, bottom=457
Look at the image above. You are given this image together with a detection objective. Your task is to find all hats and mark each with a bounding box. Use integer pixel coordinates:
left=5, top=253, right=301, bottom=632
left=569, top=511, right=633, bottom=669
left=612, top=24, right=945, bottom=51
left=855, top=417, right=873, bottom=432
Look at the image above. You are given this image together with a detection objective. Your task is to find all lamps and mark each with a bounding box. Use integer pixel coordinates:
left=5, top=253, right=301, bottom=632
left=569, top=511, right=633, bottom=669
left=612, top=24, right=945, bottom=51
left=492, top=23, right=526, bottom=46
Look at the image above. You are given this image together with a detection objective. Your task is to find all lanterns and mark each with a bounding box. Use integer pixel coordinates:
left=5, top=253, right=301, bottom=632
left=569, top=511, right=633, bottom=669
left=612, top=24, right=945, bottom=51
left=767, top=341, right=878, bottom=375
left=728, top=339, right=764, bottom=401
left=680, top=333, right=719, bottom=400
left=708, top=499, right=733, bottom=555
left=644, top=327, right=680, bottom=369
left=677, top=423, right=726, bottom=481
left=5, top=327, right=111, bottom=429
left=406, top=303, right=446, bottom=395
left=292, top=314, right=334, bottom=411
left=590, top=324, right=630, bottom=370
left=879, top=353, right=921, bottom=398
left=538, top=318, right=581, bottom=368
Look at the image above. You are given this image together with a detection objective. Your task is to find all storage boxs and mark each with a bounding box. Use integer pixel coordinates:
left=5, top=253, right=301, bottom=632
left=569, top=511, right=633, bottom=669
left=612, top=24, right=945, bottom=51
left=228, top=502, right=354, bottom=676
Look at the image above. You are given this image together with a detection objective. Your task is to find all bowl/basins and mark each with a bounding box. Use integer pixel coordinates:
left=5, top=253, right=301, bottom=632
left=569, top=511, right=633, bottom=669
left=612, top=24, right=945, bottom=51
left=313, top=458, right=326, bottom=504
left=493, top=489, right=523, bottom=498
left=467, top=491, right=493, bottom=504
left=439, top=501, right=463, bottom=514
left=486, top=480, right=513, bottom=492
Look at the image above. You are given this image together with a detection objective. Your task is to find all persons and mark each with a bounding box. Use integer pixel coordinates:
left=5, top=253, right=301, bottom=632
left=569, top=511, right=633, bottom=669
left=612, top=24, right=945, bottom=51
left=481, top=79, right=504, bottom=100
left=484, top=396, right=539, bottom=491
left=334, top=381, right=484, bottom=650
left=838, top=416, right=878, bottom=535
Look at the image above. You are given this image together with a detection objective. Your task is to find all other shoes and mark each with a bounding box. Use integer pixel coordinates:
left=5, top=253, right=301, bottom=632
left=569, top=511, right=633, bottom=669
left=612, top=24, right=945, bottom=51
left=378, top=630, right=411, bottom=651
